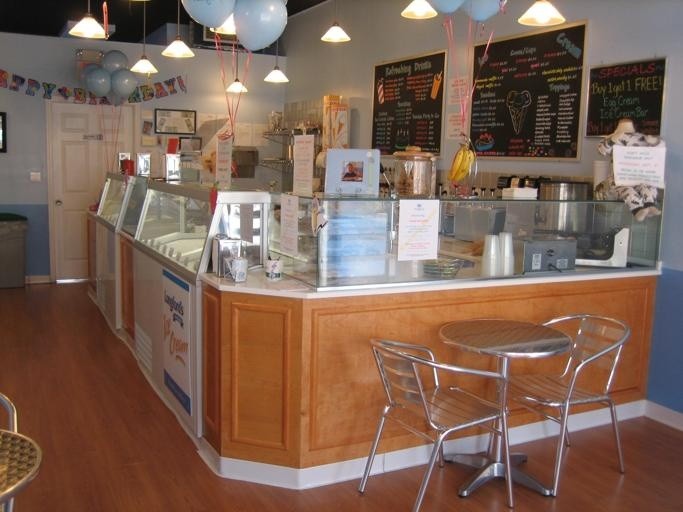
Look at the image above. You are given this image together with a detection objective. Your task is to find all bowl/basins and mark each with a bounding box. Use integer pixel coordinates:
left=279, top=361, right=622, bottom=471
left=420, top=256, right=463, bottom=280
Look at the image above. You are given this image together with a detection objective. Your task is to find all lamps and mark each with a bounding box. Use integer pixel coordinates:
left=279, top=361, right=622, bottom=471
left=130, top=1, right=160, bottom=75
left=401, top=1, right=438, bottom=20
left=319, top=1, right=351, bottom=44
left=158, top=0, right=196, bottom=59
left=223, top=39, right=250, bottom=94
left=67, top=1, right=110, bottom=40
left=263, top=40, right=290, bottom=84
left=517, top=1, right=566, bottom=27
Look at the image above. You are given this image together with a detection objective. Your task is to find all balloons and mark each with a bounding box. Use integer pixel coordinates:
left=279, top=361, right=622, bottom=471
left=232, top=0, right=288, bottom=52
left=177, top=0, right=289, bottom=36
left=78, top=48, right=139, bottom=109
left=427, top=0, right=506, bottom=148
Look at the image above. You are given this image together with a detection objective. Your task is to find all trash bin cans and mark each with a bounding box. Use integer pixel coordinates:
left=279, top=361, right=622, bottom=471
left=0, top=212, right=29, bottom=289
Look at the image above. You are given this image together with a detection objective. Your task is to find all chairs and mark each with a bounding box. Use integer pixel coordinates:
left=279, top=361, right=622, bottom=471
left=0, top=388, right=26, bottom=512
left=358, top=334, right=516, bottom=511
left=485, top=304, right=631, bottom=496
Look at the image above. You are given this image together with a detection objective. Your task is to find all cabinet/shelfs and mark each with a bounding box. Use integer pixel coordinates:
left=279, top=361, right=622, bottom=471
left=257, top=92, right=350, bottom=195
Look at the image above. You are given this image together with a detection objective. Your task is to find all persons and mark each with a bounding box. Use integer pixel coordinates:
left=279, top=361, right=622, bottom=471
left=341, top=160, right=362, bottom=182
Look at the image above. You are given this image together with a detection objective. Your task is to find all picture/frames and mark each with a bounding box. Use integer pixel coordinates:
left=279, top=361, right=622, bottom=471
left=176, top=136, right=203, bottom=153
left=322, top=145, right=382, bottom=198
left=152, top=107, right=198, bottom=137
left=0, top=109, right=10, bottom=153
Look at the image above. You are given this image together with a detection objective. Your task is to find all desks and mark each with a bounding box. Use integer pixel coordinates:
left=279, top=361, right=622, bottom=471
left=0, top=422, right=46, bottom=506
left=435, top=318, right=574, bottom=500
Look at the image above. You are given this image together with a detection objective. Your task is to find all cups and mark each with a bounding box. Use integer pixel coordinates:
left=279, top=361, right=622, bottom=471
left=374, top=80, right=385, bottom=104
left=428, top=73, right=441, bottom=99
left=229, top=258, right=249, bottom=282
left=482, top=231, right=515, bottom=278
left=119, top=159, right=134, bottom=176
left=263, top=259, right=283, bottom=281
